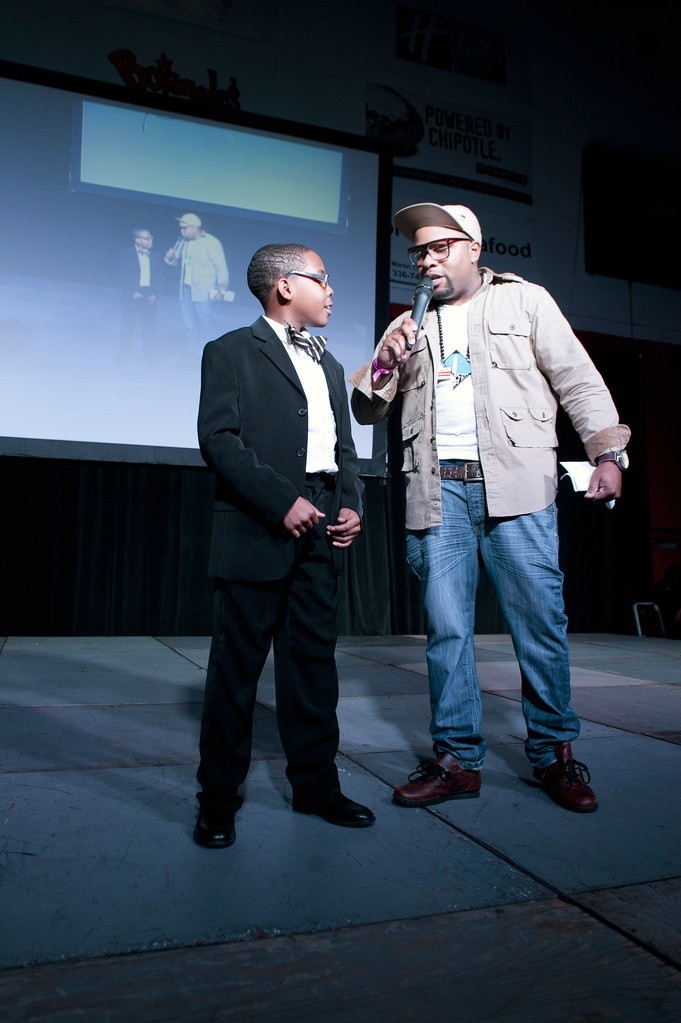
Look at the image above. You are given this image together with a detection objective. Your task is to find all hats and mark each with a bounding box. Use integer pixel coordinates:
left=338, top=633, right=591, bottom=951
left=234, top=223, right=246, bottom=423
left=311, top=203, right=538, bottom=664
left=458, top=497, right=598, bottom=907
left=394, top=203, right=482, bottom=248
left=174, top=212, right=201, bottom=228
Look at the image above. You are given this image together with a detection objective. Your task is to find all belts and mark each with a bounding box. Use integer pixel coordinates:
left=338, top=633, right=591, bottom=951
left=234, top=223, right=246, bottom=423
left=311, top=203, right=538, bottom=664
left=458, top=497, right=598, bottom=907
left=440, top=462, right=484, bottom=483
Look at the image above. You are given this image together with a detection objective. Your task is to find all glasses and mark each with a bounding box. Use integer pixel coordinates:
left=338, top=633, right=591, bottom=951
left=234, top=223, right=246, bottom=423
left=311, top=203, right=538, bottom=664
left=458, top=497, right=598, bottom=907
left=283, top=272, right=330, bottom=289
left=135, top=235, right=154, bottom=241
left=407, top=238, right=473, bottom=266
left=179, top=225, right=192, bottom=230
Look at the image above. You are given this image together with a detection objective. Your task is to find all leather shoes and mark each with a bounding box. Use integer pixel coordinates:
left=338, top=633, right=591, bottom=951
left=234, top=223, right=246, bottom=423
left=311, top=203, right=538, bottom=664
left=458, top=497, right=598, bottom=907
left=192, top=799, right=236, bottom=847
left=533, top=741, right=598, bottom=814
left=291, top=788, right=376, bottom=827
left=393, top=753, right=482, bottom=807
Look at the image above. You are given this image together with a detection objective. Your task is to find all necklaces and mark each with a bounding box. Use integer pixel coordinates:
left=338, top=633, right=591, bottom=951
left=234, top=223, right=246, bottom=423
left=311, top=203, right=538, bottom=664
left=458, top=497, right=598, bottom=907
left=434, top=301, right=471, bottom=381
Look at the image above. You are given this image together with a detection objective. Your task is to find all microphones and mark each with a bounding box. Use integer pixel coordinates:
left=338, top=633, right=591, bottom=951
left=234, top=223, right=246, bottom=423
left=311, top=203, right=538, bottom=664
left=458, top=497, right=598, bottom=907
left=397, top=274, right=436, bottom=391
left=172, top=236, right=183, bottom=251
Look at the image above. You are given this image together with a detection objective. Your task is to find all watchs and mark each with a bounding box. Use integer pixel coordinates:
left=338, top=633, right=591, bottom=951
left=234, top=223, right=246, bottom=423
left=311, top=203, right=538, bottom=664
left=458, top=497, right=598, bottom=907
left=596, top=450, right=629, bottom=470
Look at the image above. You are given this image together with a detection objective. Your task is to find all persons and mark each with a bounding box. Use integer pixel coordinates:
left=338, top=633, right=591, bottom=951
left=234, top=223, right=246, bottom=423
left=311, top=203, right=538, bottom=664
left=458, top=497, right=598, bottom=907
left=166, top=213, right=230, bottom=326
left=191, top=244, right=377, bottom=850
left=349, top=202, right=631, bottom=813
left=122, top=227, right=163, bottom=314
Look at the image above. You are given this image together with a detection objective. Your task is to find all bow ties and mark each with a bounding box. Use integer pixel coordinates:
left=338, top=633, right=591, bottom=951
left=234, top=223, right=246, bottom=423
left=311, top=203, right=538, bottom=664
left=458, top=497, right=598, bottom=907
left=284, top=320, right=328, bottom=361
left=138, top=247, right=151, bottom=256
